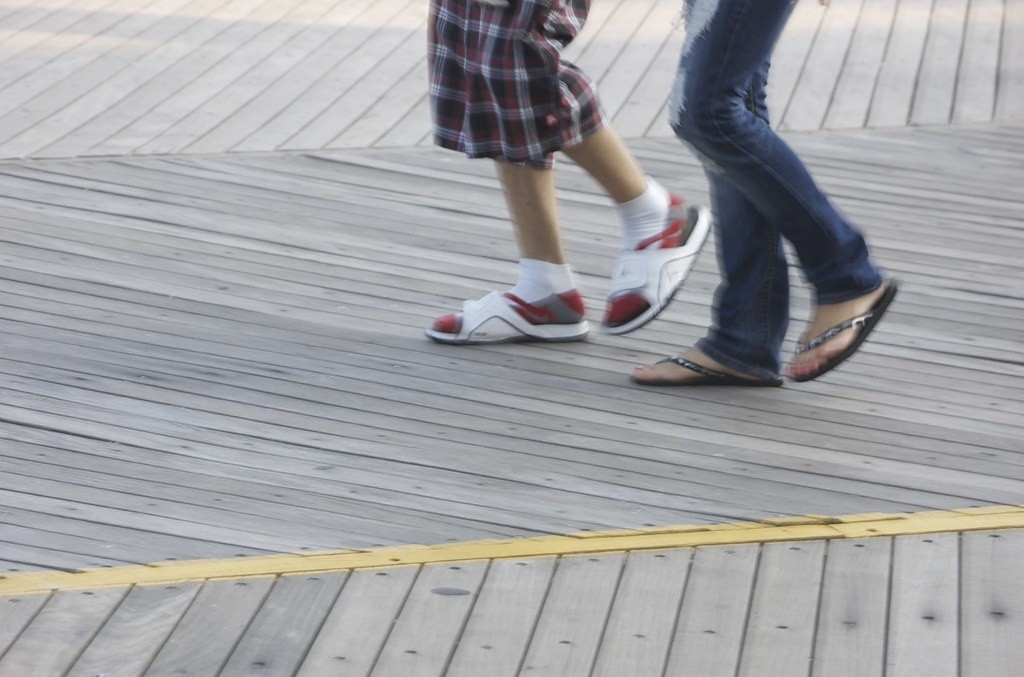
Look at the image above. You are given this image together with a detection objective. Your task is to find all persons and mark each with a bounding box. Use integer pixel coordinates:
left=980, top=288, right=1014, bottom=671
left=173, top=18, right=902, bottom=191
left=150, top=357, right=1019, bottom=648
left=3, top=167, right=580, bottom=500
left=631, top=0, right=900, bottom=389
left=418, top=0, right=712, bottom=344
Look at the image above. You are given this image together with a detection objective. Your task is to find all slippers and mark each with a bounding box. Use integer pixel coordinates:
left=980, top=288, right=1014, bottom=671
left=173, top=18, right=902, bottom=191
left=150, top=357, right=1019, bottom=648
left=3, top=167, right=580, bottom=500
left=425, top=291, right=590, bottom=345
left=629, top=356, right=784, bottom=387
left=786, top=278, right=898, bottom=381
left=599, top=204, right=712, bottom=335
